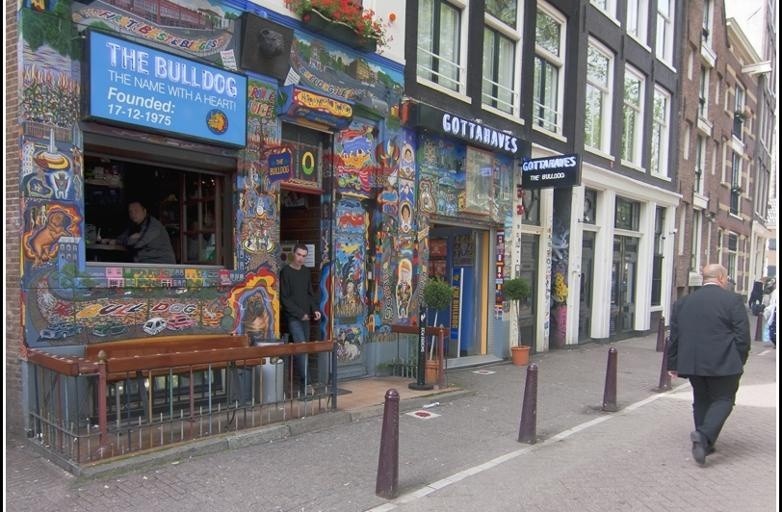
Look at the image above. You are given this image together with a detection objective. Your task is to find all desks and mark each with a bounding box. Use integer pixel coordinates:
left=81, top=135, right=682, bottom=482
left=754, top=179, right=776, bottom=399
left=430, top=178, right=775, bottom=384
left=86, top=244, right=130, bottom=262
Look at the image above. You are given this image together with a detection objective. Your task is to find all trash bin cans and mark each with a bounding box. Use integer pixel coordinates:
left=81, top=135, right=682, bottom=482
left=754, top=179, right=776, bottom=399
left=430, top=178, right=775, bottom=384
left=256, top=341, right=284, bottom=404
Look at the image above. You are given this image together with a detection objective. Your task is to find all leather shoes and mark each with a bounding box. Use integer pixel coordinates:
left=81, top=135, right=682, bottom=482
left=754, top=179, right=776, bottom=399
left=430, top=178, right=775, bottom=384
left=690, top=428, right=713, bottom=465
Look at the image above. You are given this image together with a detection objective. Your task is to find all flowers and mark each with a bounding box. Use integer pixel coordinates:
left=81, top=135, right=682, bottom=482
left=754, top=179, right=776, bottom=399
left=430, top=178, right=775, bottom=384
left=284, top=0, right=396, bottom=54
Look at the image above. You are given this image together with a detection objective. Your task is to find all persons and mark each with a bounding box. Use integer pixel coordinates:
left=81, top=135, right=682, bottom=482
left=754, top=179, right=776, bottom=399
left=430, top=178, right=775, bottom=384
left=278, top=242, right=323, bottom=394
left=664, top=263, right=753, bottom=465
left=399, top=204, right=412, bottom=223
left=762, top=289, right=775, bottom=349
left=108, top=200, right=177, bottom=265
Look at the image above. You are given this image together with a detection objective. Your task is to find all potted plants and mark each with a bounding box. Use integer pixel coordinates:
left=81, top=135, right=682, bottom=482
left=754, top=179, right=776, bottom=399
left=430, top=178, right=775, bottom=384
left=502, top=278, right=531, bottom=367
left=424, top=277, right=456, bottom=384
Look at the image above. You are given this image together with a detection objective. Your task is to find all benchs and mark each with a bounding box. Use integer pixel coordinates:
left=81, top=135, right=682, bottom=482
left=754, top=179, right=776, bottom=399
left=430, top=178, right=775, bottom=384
left=84, top=335, right=266, bottom=424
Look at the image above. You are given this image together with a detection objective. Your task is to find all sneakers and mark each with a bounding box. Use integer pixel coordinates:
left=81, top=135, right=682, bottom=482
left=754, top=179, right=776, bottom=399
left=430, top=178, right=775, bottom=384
left=300, top=383, right=316, bottom=395
